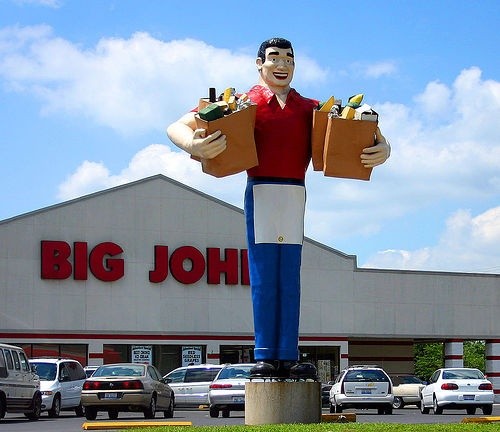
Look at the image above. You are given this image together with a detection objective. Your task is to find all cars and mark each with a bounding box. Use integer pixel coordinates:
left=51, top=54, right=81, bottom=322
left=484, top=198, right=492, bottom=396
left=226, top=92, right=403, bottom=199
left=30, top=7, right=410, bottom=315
left=83, top=365, right=102, bottom=379
left=206, top=363, right=258, bottom=418
left=391, top=375, right=428, bottom=409
left=321, top=364, right=395, bottom=416
left=80, top=362, right=176, bottom=420
left=418, top=366, right=494, bottom=415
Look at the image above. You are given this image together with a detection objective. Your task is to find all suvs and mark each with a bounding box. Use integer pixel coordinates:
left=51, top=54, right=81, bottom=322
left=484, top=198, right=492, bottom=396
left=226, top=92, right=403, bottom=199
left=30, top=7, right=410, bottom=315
left=0, top=343, right=43, bottom=422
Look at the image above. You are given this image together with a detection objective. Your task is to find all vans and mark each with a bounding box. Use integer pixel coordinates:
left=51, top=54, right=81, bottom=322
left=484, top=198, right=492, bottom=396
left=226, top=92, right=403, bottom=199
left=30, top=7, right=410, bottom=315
left=15, top=357, right=89, bottom=418
left=162, top=363, right=226, bottom=410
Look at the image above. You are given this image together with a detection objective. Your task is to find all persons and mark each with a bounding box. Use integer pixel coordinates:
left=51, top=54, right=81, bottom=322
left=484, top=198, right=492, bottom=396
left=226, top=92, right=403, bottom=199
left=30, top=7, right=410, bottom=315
left=167, top=37, right=391, bottom=377
